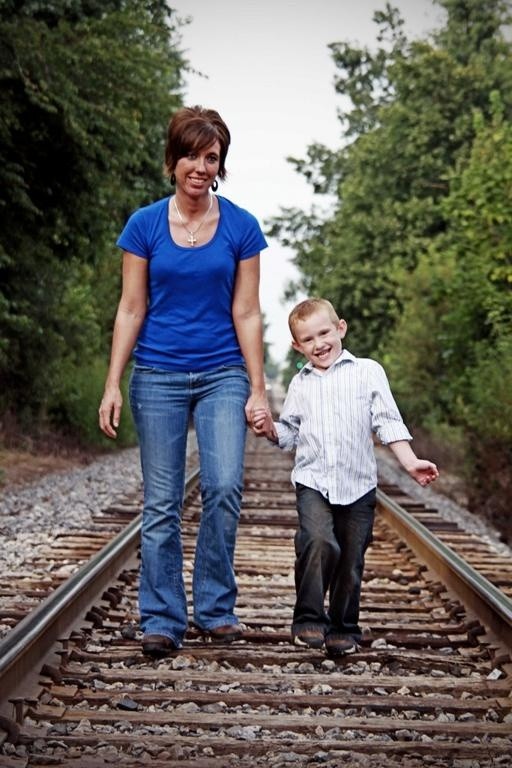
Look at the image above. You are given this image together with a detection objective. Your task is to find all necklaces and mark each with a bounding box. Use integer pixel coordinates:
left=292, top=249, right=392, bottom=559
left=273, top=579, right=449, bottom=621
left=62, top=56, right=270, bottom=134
left=173, top=192, right=214, bottom=246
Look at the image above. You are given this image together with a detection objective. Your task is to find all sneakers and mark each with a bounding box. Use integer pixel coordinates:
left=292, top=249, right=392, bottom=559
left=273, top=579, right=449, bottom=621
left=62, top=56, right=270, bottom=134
left=294, top=628, right=326, bottom=648
left=208, top=624, right=243, bottom=638
left=325, top=634, right=357, bottom=656
left=141, top=633, right=177, bottom=655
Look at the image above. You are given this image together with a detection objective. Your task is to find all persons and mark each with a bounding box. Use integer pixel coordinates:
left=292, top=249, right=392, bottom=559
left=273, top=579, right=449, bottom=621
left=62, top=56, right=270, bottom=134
left=97, top=105, right=275, bottom=655
left=252, top=295, right=441, bottom=657
